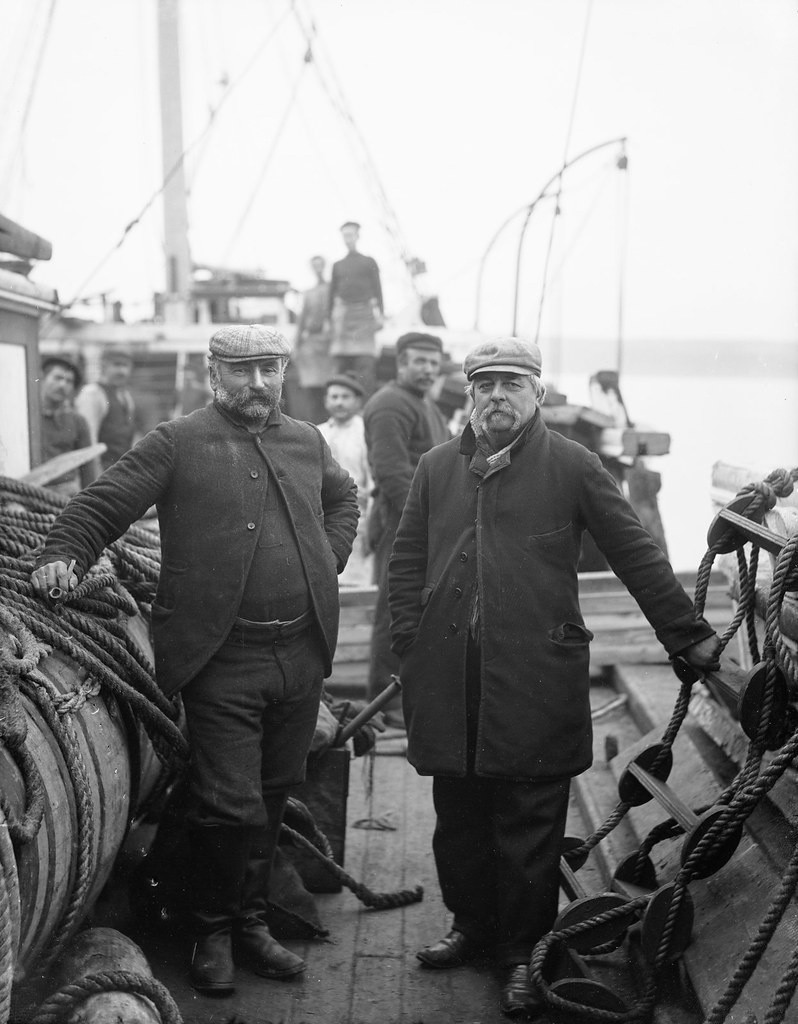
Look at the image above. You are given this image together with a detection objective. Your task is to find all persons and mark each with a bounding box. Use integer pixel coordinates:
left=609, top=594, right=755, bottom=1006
left=36, top=350, right=100, bottom=496
left=170, top=362, right=216, bottom=416
left=315, top=370, right=379, bottom=586
left=390, top=339, right=723, bottom=1018
left=362, top=332, right=456, bottom=587
left=293, top=254, right=332, bottom=421
left=76, top=343, right=137, bottom=481
left=36, top=328, right=361, bottom=996
left=327, top=221, right=383, bottom=386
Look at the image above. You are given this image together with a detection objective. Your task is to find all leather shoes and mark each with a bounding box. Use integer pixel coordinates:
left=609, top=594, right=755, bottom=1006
left=415, top=929, right=469, bottom=968
left=500, top=964, right=541, bottom=1016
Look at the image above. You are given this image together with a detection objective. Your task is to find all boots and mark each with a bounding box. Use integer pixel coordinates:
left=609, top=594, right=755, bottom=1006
left=233, top=831, right=307, bottom=977
left=183, top=823, right=252, bottom=993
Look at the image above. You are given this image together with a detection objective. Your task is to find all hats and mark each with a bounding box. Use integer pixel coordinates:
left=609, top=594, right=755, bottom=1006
left=42, top=356, right=82, bottom=387
left=340, top=221, right=361, bottom=231
left=327, top=370, right=363, bottom=395
left=462, top=337, right=541, bottom=382
left=99, top=348, right=135, bottom=359
left=208, top=325, right=291, bottom=362
left=396, top=332, right=443, bottom=354
